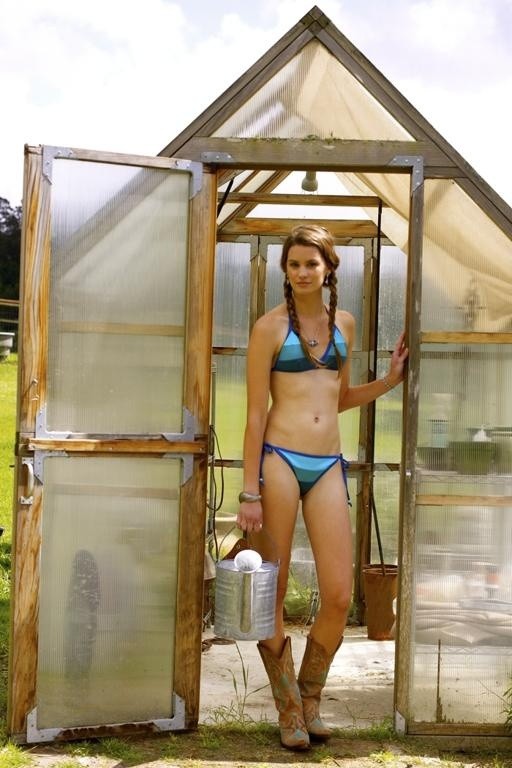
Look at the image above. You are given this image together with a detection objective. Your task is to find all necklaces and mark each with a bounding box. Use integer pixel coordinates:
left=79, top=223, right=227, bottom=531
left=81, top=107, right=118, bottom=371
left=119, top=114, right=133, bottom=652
left=297, top=304, right=323, bottom=347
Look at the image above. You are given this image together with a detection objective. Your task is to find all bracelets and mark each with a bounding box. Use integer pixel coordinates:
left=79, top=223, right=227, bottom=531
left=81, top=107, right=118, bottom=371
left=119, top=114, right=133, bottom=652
left=383, top=375, right=395, bottom=390
left=238, top=492, right=262, bottom=504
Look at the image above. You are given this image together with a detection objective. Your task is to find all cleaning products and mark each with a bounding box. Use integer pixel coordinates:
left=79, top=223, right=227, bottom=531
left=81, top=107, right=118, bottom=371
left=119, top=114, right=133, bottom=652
left=428, top=407, right=451, bottom=446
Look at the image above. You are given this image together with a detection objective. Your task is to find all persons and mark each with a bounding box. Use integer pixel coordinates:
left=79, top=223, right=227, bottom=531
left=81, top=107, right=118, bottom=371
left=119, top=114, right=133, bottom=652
left=233, top=224, right=409, bottom=751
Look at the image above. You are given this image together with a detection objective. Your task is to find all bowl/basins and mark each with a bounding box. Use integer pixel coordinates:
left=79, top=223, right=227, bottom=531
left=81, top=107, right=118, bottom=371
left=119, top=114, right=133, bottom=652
left=416, top=442, right=496, bottom=475
